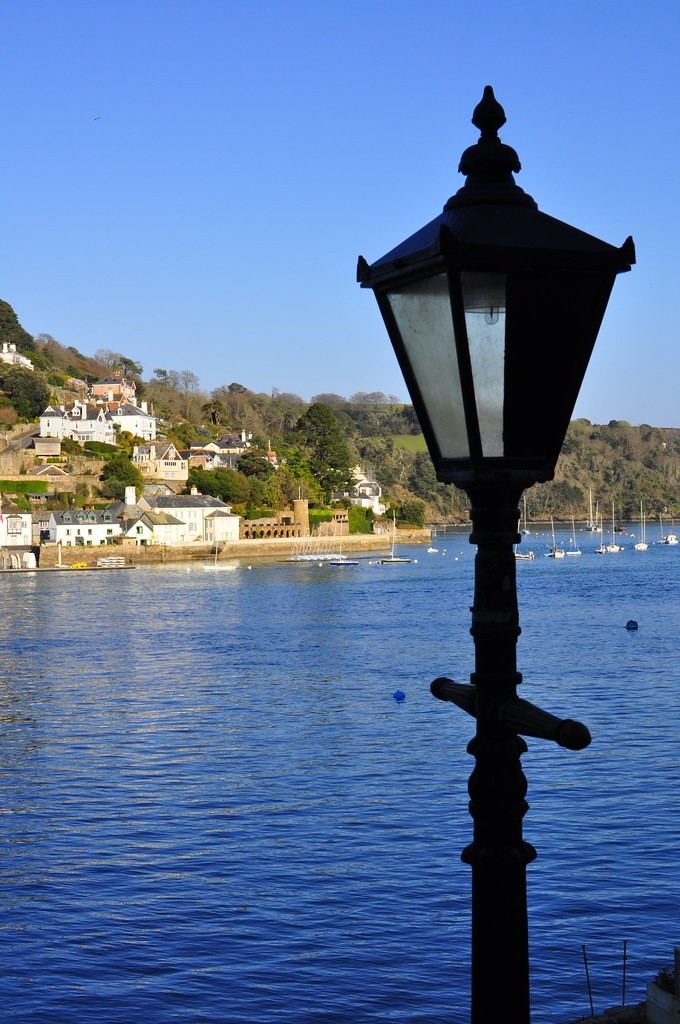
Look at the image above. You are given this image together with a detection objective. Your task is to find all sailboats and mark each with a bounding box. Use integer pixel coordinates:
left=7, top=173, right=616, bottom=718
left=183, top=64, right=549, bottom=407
left=586, top=487, right=679, bottom=555
left=566, top=515, right=582, bottom=555
left=544, top=515, right=565, bottom=558
left=381, top=509, right=411, bottom=564
left=202, top=536, right=236, bottom=570
left=513, top=519, right=534, bottom=560
left=522, top=495, right=531, bottom=535
left=329, top=518, right=358, bottom=565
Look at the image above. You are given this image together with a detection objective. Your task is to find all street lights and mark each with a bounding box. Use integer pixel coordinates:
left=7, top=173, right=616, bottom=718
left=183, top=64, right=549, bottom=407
left=357, top=85, right=638, bottom=1023
